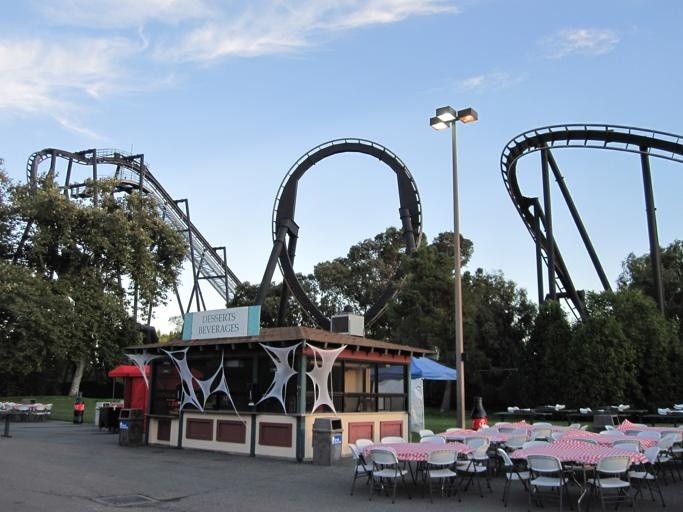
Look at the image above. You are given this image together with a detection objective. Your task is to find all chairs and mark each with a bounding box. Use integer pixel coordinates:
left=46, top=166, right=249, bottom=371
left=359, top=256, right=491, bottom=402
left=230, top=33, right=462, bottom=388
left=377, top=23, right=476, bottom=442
left=346, top=422, right=683, bottom=512
left=0, top=401, right=53, bottom=422
left=493, top=404, right=682, bottom=427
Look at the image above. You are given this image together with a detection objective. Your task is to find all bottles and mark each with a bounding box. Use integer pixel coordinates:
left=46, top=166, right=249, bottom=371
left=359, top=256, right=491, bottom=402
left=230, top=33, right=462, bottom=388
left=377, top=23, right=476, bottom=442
left=73, top=392, right=84, bottom=424
left=471, top=396, right=488, bottom=431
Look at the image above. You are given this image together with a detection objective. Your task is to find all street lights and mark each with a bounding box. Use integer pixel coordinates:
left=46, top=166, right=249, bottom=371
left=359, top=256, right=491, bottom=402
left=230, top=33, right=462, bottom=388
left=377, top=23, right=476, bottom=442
left=430, top=105, right=478, bottom=428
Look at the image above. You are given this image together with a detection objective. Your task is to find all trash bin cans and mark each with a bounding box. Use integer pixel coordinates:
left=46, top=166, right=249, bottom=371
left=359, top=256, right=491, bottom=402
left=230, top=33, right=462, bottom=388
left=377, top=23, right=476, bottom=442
left=593, top=415, right=620, bottom=433
left=312, top=417, right=343, bottom=465
left=119, top=409, right=145, bottom=446
left=74, top=403, right=84, bottom=423
